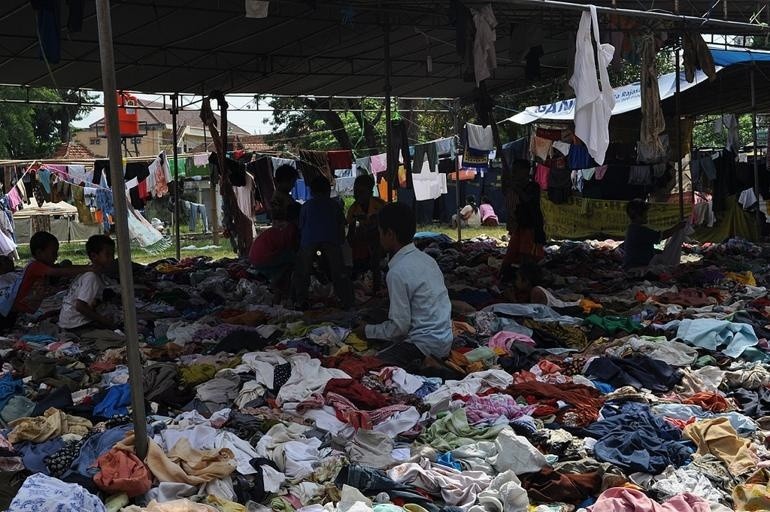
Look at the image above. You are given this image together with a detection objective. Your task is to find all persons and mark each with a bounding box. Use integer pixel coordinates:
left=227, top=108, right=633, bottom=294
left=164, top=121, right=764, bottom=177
left=270, top=164, right=302, bottom=291
left=504, top=182, right=544, bottom=284
left=347, top=176, right=388, bottom=265
left=625, top=200, right=686, bottom=270
left=353, top=203, right=468, bottom=380
left=493, top=159, right=531, bottom=294
left=503, top=264, right=582, bottom=310
left=14, top=230, right=74, bottom=319
left=56, top=233, right=125, bottom=350
left=479, top=196, right=498, bottom=226
left=296, top=177, right=352, bottom=308
left=451, top=195, right=481, bottom=231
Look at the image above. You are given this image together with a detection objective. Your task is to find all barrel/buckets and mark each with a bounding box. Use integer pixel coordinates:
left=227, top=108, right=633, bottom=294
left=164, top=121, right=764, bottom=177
left=104, top=90, right=138, bottom=134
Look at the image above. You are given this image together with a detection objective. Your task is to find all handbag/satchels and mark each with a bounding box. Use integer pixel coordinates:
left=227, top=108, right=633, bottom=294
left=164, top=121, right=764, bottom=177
left=462, top=211, right=473, bottom=220
